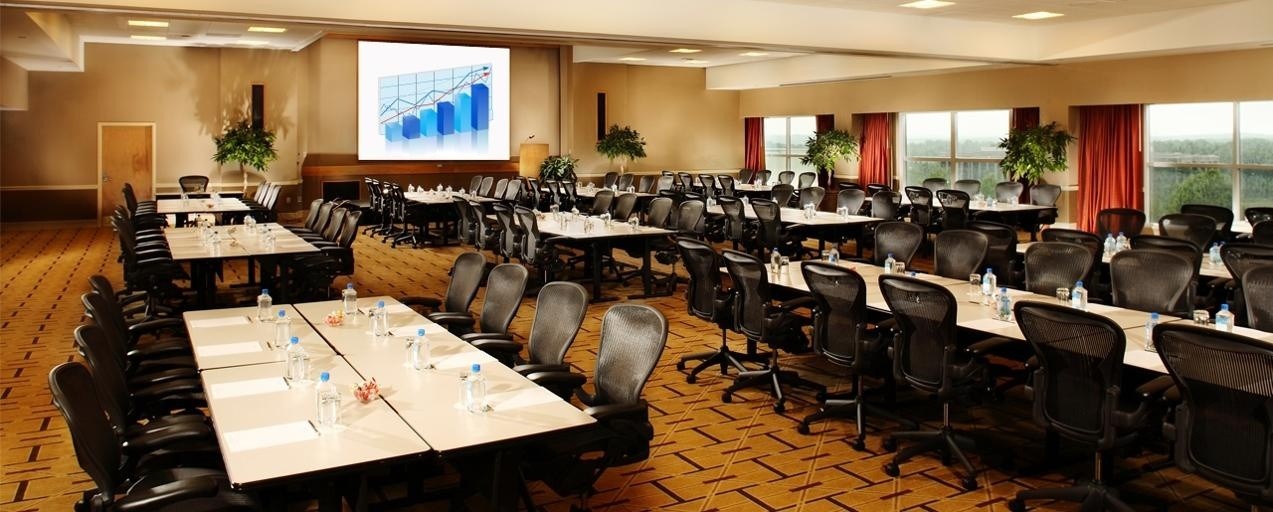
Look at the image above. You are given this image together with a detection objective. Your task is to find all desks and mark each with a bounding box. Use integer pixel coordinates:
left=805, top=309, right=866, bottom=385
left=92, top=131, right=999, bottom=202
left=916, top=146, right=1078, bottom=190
left=480, top=208, right=680, bottom=304
left=155, top=198, right=323, bottom=293
left=702, top=199, right=884, bottom=257
left=180, top=294, right=596, bottom=512
left=715, top=261, right=1273, bottom=450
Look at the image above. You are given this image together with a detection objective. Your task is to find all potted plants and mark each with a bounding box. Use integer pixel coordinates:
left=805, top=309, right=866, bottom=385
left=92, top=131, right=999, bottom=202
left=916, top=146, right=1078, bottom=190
left=798, top=129, right=863, bottom=213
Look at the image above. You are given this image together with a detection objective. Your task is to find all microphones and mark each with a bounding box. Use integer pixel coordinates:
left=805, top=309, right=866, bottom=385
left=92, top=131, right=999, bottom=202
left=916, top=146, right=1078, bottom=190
left=523, top=135, right=536, bottom=143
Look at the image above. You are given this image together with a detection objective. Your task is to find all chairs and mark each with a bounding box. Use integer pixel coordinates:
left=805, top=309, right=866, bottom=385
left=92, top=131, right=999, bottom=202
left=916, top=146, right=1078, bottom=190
left=105, top=178, right=181, bottom=317
left=795, top=260, right=895, bottom=452
left=675, top=170, right=1273, bottom=336
left=877, top=274, right=1039, bottom=489
left=393, top=250, right=670, bottom=512
left=361, top=170, right=676, bottom=296
left=239, top=178, right=362, bottom=286
left=1151, top=321, right=1272, bottom=511
left=720, top=248, right=815, bottom=415
left=48, top=274, right=238, bottom=512
left=676, top=167, right=1271, bottom=509
left=1008, top=297, right=1176, bottom=510
left=673, top=236, right=770, bottom=385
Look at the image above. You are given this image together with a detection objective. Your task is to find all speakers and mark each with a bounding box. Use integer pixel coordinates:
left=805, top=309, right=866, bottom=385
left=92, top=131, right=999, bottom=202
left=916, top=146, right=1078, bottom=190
left=251, top=83, right=264, bottom=131
left=597, top=91, right=607, bottom=141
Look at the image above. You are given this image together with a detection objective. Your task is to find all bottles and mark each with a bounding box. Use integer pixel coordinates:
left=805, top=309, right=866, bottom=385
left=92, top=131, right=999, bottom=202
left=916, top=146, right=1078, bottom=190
left=405, top=181, right=480, bottom=204
left=884, top=254, right=895, bottom=276
left=1073, top=281, right=1088, bottom=312
left=973, top=191, right=1021, bottom=207
left=769, top=248, right=780, bottom=273
left=522, top=204, right=643, bottom=233
left=1216, top=303, right=1235, bottom=333
left=1143, top=312, right=1161, bottom=352
left=569, top=176, right=851, bottom=221
left=1102, top=231, right=1228, bottom=265
left=999, top=288, right=1011, bottom=320
left=176, top=189, right=278, bottom=250
left=892, top=176, right=900, bottom=192
left=829, top=247, right=840, bottom=263
left=777, top=256, right=790, bottom=282
left=250, top=282, right=493, bottom=422
left=984, top=268, right=997, bottom=305
